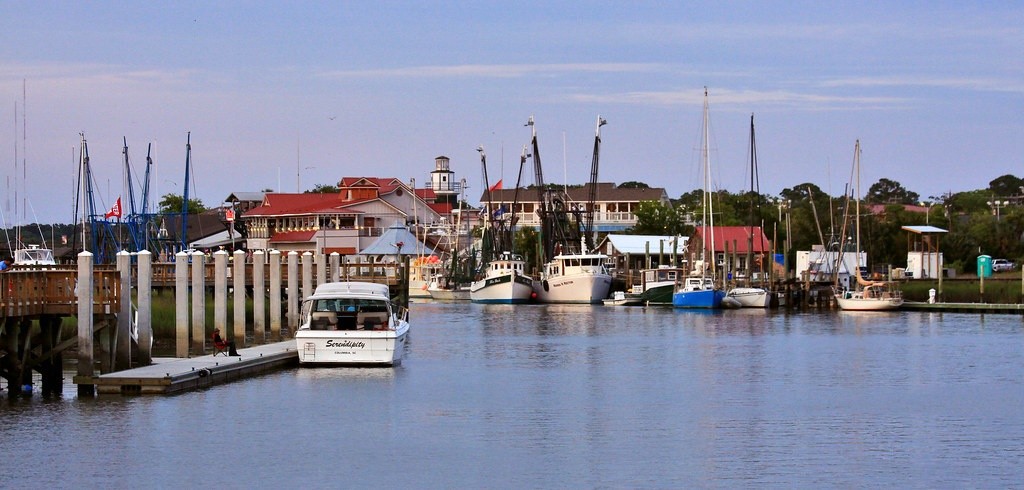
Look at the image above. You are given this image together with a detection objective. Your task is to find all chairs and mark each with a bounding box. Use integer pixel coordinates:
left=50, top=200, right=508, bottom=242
left=357, top=313, right=388, bottom=329
left=312, top=312, right=339, bottom=330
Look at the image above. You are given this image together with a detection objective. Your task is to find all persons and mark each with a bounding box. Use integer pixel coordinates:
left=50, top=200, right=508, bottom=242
left=0, top=257, right=14, bottom=299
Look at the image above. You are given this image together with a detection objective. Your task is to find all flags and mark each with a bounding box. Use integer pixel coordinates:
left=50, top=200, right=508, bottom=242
left=490, top=179, right=502, bottom=192
left=494, top=206, right=505, bottom=217
left=104, top=198, right=121, bottom=219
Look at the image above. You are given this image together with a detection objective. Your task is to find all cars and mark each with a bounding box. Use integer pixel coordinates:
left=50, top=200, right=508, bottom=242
left=991, top=259, right=1015, bottom=272
left=295, top=281, right=410, bottom=365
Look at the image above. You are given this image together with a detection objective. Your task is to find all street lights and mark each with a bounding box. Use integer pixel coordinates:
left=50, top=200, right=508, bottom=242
left=987, top=200, right=1011, bottom=221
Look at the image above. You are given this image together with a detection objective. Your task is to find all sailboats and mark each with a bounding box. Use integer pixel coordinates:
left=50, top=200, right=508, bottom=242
left=406, top=176, right=435, bottom=298
left=525, top=112, right=615, bottom=304
left=729, top=114, right=775, bottom=309
left=671, top=83, right=727, bottom=310
left=427, top=175, right=475, bottom=300
left=470, top=142, right=534, bottom=304
left=829, top=140, right=904, bottom=312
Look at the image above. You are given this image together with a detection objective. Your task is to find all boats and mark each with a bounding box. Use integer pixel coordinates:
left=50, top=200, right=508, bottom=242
left=602, top=276, right=679, bottom=306
